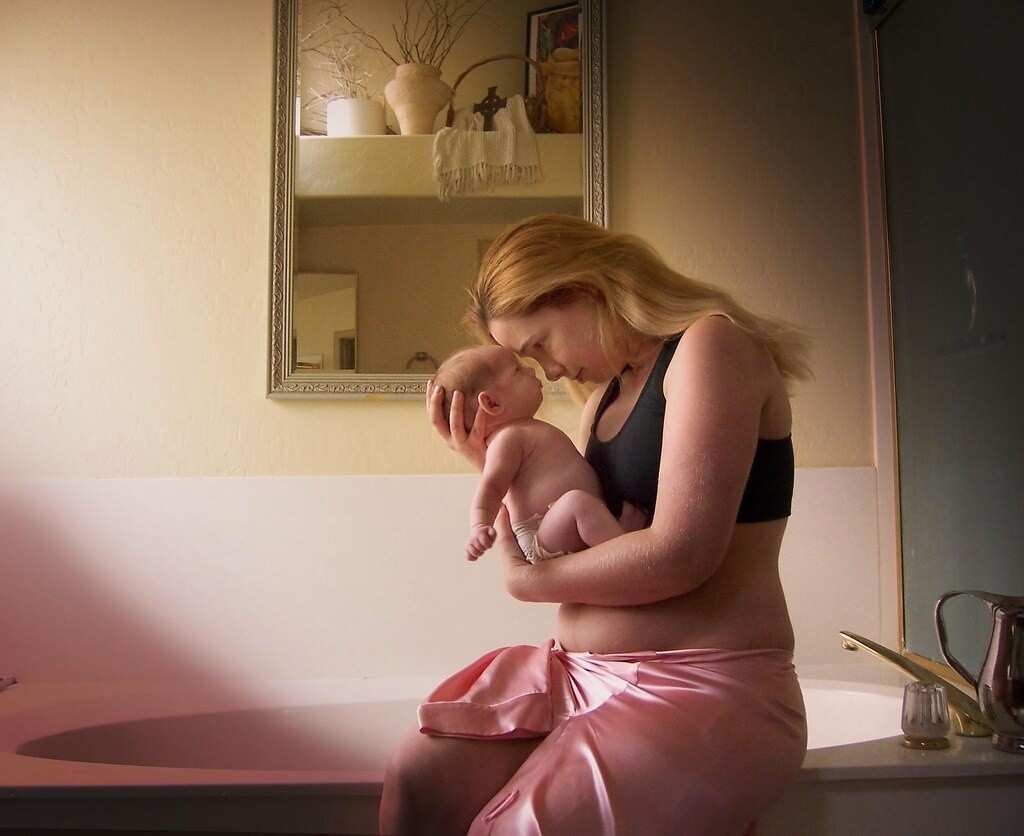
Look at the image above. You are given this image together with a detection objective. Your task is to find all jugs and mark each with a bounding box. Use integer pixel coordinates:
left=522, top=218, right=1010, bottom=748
left=934, top=589, right=1024, bottom=753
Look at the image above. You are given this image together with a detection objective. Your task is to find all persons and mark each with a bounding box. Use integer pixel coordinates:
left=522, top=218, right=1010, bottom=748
left=380, top=214, right=806, bottom=836
left=431, top=346, right=647, bottom=564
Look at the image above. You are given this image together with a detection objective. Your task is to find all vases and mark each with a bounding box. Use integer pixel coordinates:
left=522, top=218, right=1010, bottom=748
left=327, top=100, right=384, bottom=134
left=383, top=63, right=453, bottom=135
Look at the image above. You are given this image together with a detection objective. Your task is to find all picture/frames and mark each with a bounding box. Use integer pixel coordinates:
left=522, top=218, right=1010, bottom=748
left=525, top=3, right=582, bottom=134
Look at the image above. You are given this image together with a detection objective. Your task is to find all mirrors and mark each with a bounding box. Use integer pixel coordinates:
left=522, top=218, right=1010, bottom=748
left=265, top=0, right=610, bottom=401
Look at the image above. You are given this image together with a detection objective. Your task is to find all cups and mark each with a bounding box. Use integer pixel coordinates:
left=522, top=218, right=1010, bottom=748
left=900, top=681, right=951, bottom=750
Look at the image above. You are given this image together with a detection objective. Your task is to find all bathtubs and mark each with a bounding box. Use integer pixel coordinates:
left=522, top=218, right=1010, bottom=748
left=0, top=676, right=1024, bottom=836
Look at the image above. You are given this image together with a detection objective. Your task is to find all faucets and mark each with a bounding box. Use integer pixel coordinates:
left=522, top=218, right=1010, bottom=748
left=839, top=630, right=995, bottom=738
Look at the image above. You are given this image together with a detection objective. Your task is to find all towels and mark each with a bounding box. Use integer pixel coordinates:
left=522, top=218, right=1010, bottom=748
left=432, top=93, right=544, bottom=206
